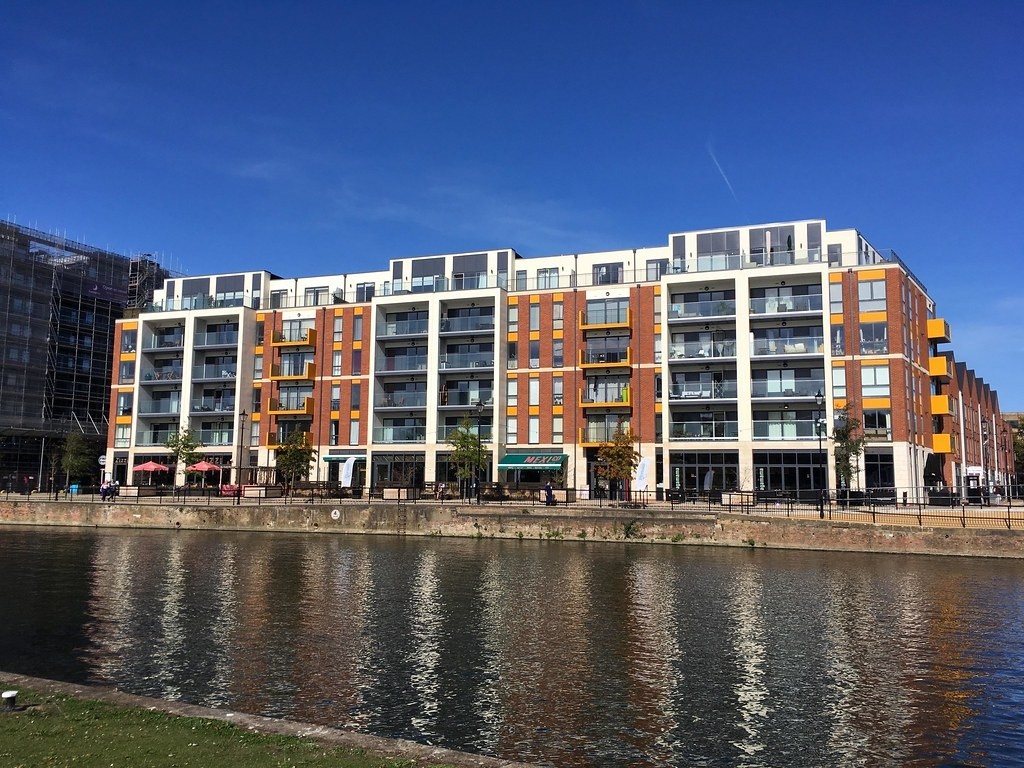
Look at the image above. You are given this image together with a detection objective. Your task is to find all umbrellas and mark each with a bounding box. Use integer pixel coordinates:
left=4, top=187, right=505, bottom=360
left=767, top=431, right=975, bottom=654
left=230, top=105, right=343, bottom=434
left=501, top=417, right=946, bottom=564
left=186, top=461, right=220, bottom=488
left=133, top=461, right=169, bottom=485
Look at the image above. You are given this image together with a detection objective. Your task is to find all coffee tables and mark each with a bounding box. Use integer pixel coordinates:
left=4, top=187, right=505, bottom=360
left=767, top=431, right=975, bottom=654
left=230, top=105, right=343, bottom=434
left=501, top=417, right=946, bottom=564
left=684, top=394, right=699, bottom=399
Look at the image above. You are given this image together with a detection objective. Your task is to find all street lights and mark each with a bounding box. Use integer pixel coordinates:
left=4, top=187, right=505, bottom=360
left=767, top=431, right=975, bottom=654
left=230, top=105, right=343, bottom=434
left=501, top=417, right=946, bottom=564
left=1001, top=428, right=1010, bottom=500
left=475, top=399, right=484, bottom=505
left=237, top=409, right=249, bottom=505
left=815, top=389, right=824, bottom=519
left=981, top=418, right=990, bottom=506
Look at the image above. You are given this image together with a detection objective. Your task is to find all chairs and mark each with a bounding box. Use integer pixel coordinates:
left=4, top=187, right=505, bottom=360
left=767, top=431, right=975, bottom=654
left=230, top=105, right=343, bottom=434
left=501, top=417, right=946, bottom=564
left=669, top=389, right=681, bottom=399
left=701, top=390, right=711, bottom=398
left=769, top=340, right=778, bottom=355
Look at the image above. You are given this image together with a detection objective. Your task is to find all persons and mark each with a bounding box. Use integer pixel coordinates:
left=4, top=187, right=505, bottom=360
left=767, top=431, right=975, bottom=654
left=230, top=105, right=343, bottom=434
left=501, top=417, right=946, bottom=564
left=545, top=481, right=552, bottom=506
left=994, top=481, right=1001, bottom=504
left=436, top=483, right=443, bottom=500
left=99, top=479, right=119, bottom=502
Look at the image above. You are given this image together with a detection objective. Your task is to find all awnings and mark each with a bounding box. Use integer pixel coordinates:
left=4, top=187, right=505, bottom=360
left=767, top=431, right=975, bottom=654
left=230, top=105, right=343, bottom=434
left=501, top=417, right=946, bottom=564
left=323, top=455, right=367, bottom=461
left=497, top=453, right=568, bottom=469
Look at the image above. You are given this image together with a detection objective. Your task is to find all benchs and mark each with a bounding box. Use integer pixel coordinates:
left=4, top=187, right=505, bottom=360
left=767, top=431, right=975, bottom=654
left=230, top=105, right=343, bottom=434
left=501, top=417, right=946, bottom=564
left=784, top=388, right=794, bottom=396
left=681, top=391, right=701, bottom=399
left=784, top=343, right=807, bottom=354
